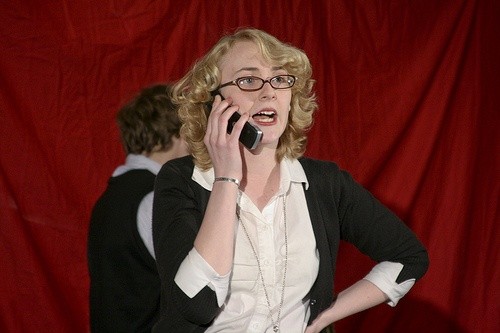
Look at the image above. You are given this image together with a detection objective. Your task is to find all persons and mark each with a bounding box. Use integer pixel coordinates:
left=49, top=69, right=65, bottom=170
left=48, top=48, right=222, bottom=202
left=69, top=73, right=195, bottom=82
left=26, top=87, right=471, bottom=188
left=152, top=27, right=430, bottom=333
left=87, top=82, right=190, bottom=333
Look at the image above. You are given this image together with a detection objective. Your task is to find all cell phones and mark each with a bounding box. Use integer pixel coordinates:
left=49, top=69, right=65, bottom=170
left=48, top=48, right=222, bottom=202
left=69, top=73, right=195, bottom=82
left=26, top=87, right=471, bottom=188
left=206, top=89, right=263, bottom=150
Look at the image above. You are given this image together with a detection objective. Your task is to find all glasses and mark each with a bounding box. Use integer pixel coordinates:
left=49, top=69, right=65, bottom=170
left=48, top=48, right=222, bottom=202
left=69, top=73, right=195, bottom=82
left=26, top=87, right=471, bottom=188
left=217, top=75, right=297, bottom=92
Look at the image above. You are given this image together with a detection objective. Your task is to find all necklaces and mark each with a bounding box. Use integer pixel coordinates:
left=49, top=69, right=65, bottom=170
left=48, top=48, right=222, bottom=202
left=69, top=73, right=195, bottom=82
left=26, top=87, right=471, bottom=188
left=236, top=197, right=288, bottom=333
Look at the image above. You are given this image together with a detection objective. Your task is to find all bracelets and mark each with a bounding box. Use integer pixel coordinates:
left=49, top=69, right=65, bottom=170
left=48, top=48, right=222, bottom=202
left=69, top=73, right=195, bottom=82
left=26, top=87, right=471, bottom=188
left=214, top=177, right=240, bottom=187
